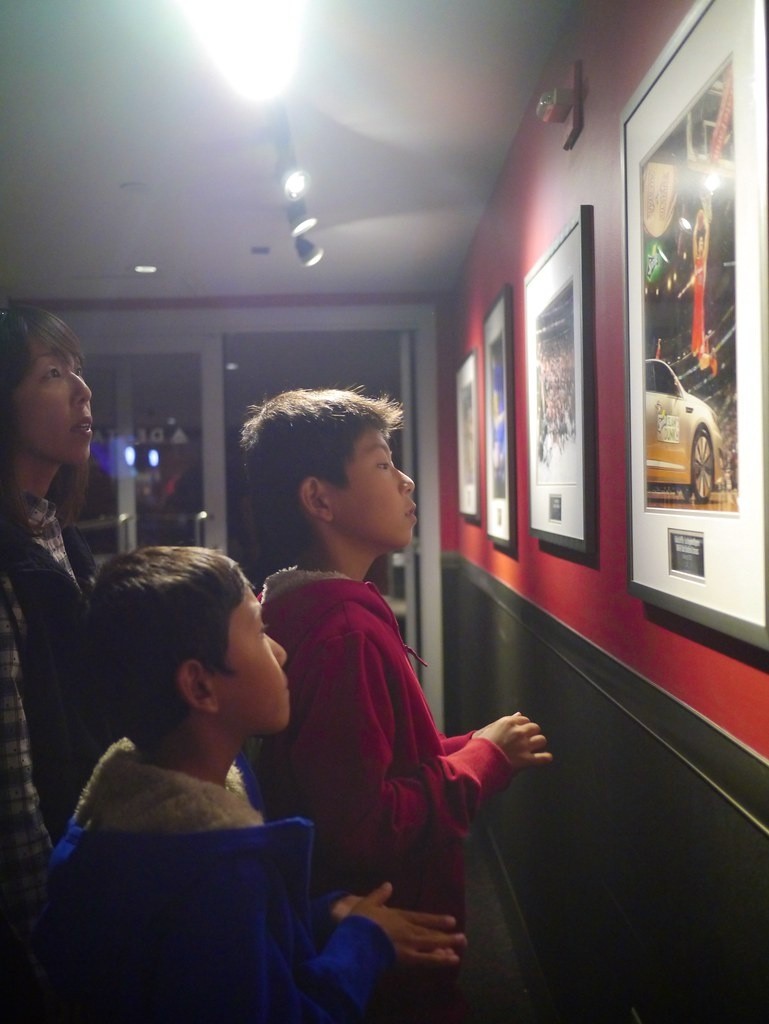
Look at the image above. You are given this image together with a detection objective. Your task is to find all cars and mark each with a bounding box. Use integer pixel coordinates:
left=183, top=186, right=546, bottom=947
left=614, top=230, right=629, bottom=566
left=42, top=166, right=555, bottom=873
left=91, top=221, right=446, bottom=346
left=645, top=358, right=726, bottom=505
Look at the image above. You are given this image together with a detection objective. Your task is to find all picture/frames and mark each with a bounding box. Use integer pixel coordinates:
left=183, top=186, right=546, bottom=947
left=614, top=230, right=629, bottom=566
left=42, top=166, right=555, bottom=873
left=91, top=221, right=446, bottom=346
left=620, top=0, right=769, bottom=652
left=456, top=347, right=481, bottom=522
left=484, top=284, right=516, bottom=550
left=523, top=204, right=594, bottom=552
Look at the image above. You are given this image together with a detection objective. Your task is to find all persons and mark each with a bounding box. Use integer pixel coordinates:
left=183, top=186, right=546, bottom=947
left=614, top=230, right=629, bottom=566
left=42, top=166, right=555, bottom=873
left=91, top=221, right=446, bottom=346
left=25, top=546, right=468, bottom=1023
left=0, top=304, right=107, bottom=1023
left=241, top=387, right=554, bottom=1023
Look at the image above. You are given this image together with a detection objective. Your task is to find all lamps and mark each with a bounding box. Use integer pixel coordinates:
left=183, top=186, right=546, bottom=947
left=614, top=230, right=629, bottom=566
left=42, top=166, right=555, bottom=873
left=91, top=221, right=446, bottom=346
left=294, top=234, right=324, bottom=267
left=282, top=168, right=310, bottom=201
left=288, top=198, right=317, bottom=234
